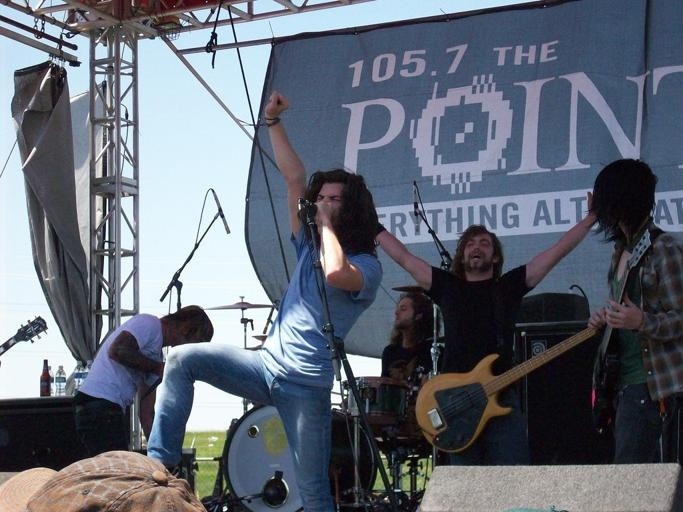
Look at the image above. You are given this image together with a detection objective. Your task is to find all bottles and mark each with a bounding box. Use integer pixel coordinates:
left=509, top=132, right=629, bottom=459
left=55, top=366, right=66, bottom=396
left=39, top=359, right=51, bottom=397
left=47, top=366, right=54, bottom=397
left=83, top=360, right=92, bottom=384
left=74, top=361, right=83, bottom=391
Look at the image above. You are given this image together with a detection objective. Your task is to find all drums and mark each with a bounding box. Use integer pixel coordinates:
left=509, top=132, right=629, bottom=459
left=222, top=403, right=380, bottom=511
left=376, top=403, right=433, bottom=453
left=344, top=378, right=411, bottom=425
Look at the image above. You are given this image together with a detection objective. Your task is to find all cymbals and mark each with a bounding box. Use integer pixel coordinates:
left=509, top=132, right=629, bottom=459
left=203, top=303, right=276, bottom=310
left=391, top=285, right=423, bottom=292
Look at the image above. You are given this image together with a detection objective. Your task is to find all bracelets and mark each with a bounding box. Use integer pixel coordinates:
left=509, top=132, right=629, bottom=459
left=265, top=116, right=280, bottom=127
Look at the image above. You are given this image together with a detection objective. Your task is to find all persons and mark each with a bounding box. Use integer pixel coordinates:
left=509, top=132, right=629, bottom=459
left=146, top=91, right=382, bottom=512
left=74, top=306, right=215, bottom=457
left=375, top=192, right=600, bottom=465
left=589, top=160, right=683, bottom=464
left=381, top=292, right=433, bottom=381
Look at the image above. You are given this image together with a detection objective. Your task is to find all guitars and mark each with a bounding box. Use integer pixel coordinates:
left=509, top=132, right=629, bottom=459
left=0, top=316, right=48, bottom=365
left=415, top=326, right=595, bottom=453
left=591, top=228, right=652, bottom=430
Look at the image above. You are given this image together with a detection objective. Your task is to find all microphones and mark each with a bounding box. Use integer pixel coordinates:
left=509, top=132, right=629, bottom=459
left=211, top=189, right=230, bottom=233
left=301, top=196, right=318, bottom=219
left=413, top=182, right=419, bottom=217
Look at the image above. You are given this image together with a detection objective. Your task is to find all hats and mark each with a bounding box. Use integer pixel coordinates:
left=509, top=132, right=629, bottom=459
left=0, top=449, right=207, bottom=512
